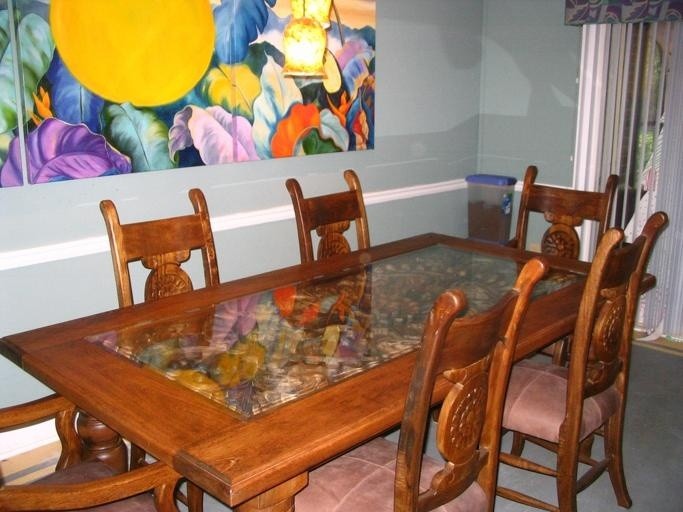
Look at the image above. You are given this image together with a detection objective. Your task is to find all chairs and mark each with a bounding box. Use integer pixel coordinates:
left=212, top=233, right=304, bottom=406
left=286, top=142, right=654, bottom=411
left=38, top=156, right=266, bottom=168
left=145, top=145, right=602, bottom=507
left=0, top=393, right=187, bottom=512
left=290, top=261, right=549, bottom=511
left=97, top=188, right=221, bottom=512
left=287, top=169, right=370, bottom=263
left=430, top=165, right=620, bottom=425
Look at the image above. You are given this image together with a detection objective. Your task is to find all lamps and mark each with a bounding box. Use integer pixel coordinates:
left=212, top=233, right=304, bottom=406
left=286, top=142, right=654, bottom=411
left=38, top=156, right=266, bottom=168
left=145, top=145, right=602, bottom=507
left=283, top=0, right=346, bottom=80
